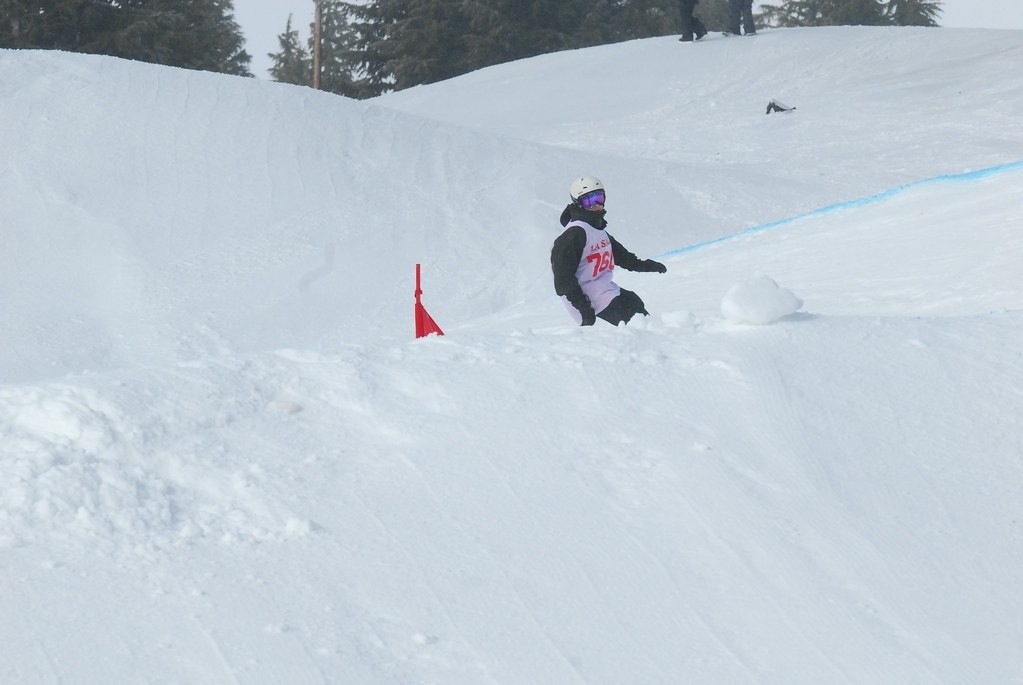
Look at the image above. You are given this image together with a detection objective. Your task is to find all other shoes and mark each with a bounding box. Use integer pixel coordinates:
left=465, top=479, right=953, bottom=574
left=742, top=32, right=756, bottom=36
left=722, top=31, right=741, bottom=37
left=678, top=37, right=693, bottom=41
left=695, top=30, right=708, bottom=40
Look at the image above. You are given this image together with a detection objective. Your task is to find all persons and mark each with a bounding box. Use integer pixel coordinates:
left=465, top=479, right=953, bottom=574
left=722, top=0, right=757, bottom=37
left=551, top=176, right=667, bottom=327
left=677, top=0, right=708, bottom=43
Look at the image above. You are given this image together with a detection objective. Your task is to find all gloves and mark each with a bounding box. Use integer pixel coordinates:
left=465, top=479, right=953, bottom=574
left=571, top=294, right=596, bottom=326
left=634, top=259, right=667, bottom=274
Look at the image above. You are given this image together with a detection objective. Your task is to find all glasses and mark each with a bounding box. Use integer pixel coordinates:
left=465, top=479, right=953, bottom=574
left=580, top=192, right=604, bottom=207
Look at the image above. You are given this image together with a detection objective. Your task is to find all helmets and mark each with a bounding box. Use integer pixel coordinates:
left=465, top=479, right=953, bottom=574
left=570, top=176, right=605, bottom=204
